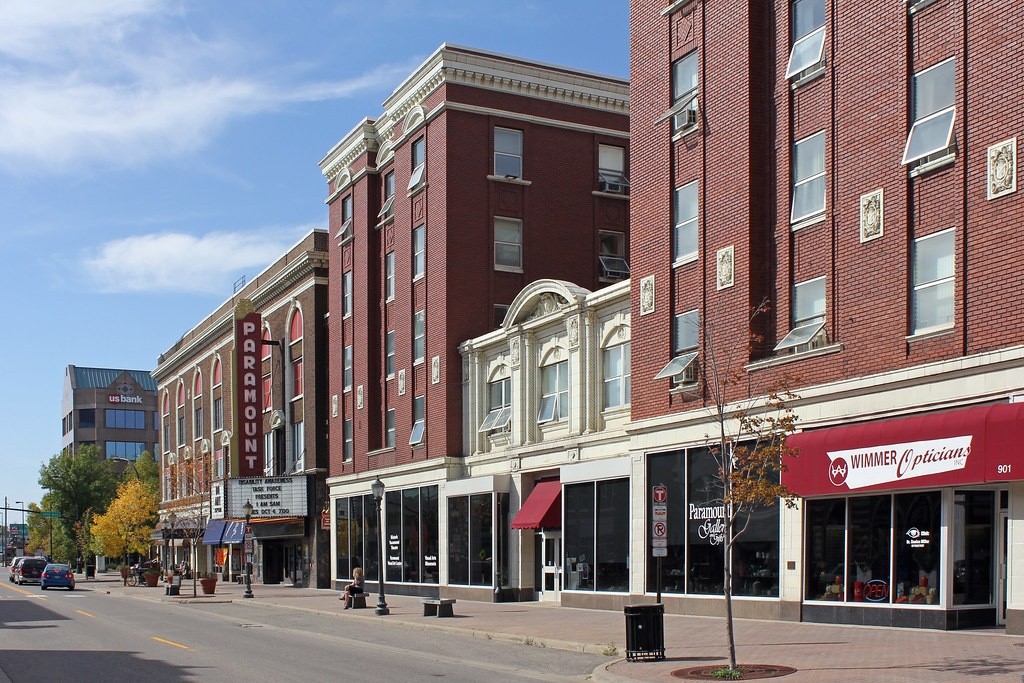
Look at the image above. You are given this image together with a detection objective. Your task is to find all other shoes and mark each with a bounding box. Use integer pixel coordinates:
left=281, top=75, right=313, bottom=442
left=339, top=597, right=345, bottom=600
left=344, top=605, right=349, bottom=609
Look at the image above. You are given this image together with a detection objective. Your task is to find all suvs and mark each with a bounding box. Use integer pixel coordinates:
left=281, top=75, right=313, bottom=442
left=14, top=556, right=47, bottom=585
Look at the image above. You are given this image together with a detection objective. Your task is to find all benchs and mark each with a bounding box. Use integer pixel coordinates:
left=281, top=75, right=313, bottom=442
left=164, top=576, right=181, bottom=596
left=341, top=592, right=369, bottom=609
left=422, top=599, right=456, bottom=618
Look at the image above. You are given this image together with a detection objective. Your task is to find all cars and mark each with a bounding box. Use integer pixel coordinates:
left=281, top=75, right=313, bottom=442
left=337, top=549, right=492, bottom=586
left=819, top=561, right=891, bottom=593
left=8, top=557, right=23, bottom=582
left=41, top=563, right=76, bottom=591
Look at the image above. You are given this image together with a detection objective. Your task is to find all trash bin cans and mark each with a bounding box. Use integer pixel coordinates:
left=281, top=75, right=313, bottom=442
left=624, top=604, right=666, bottom=661
left=87, top=565, right=94, bottom=576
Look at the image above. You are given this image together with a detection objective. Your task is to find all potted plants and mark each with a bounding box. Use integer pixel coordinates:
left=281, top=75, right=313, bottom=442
left=199, top=571, right=218, bottom=595
left=141, top=569, right=161, bottom=587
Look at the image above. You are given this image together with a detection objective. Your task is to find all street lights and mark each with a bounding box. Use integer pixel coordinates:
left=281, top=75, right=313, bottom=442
left=112, top=457, right=140, bottom=480
left=52, top=475, right=80, bottom=573
left=166, top=510, right=176, bottom=569
left=41, top=487, right=52, bottom=563
left=370, top=476, right=390, bottom=614
left=16, top=502, right=24, bottom=556
left=242, top=499, right=255, bottom=599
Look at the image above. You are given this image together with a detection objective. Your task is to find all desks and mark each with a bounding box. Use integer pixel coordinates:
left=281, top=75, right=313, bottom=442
left=472, top=560, right=492, bottom=584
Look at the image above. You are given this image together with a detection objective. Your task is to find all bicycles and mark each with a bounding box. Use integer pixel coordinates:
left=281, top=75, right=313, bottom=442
left=126, top=564, right=152, bottom=587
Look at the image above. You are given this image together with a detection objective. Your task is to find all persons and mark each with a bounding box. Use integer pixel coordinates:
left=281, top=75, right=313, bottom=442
left=338, top=568, right=365, bottom=609
left=178, top=561, right=188, bottom=576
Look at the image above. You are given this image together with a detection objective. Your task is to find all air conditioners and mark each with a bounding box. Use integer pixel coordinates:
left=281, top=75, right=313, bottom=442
left=599, top=180, right=621, bottom=194
left=675, top=109, right=697, bottom=131
left=602, top=266, right=623, bottom=279
left=795, top=334, right=824, bottom=353
left=673, top=365, right=694, bottom=383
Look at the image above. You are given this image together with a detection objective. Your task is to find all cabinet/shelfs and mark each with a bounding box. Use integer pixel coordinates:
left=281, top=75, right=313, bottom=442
left=690, top=545, right=721, bottom=592
left=741, top=544, right=779, bottom=596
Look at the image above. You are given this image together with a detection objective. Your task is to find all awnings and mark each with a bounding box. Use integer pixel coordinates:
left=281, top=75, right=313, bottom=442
left=511, top=480, right=560, bottom=529
left=221, top=520, right=246, bottom=543
left=202, top=519, right=227, bottom=544
